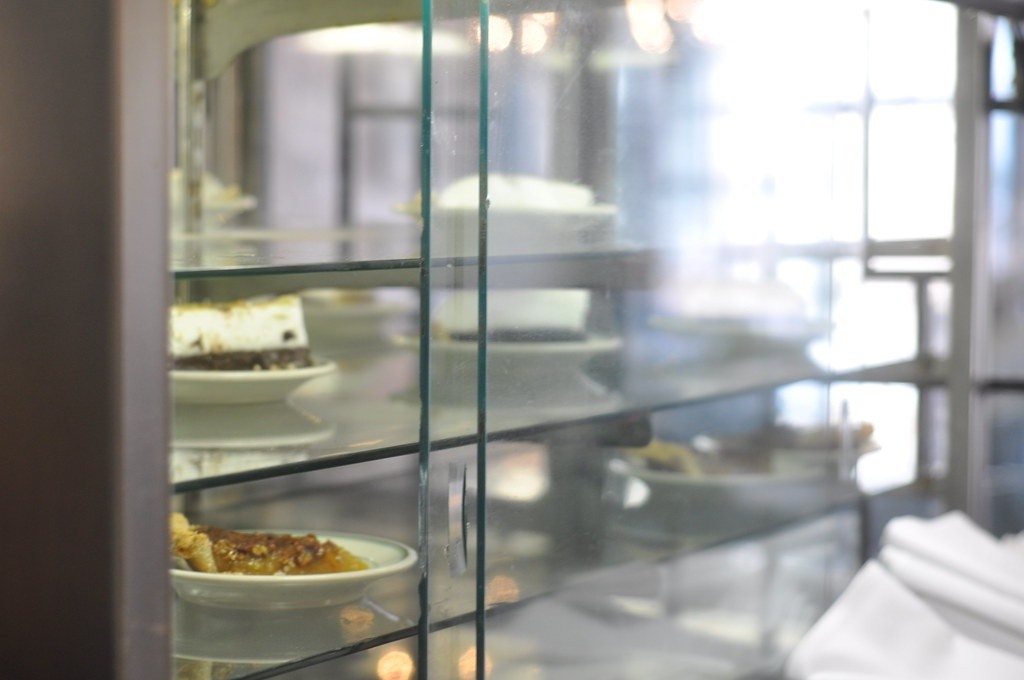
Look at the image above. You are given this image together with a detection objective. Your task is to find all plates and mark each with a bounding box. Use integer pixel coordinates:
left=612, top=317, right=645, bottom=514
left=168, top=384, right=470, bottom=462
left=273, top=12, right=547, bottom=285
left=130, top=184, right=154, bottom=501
left=641, top=311, right=835, bottom=350
left=395, top=333, right=625, bottom=370
left=169, top=527, right=417, bottom=609
left=173, top=196, right=257, bottom=230
left=169, top=352, right=337, bottom=404
left=394, top=198, right=616, bottom=245
left=610, top=455, right=828, bottom=510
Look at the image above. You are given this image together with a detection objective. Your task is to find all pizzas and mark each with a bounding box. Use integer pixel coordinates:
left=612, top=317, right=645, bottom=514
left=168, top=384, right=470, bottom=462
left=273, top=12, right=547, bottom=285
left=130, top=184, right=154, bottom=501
left=173, top=510, right=338, bottom=577
